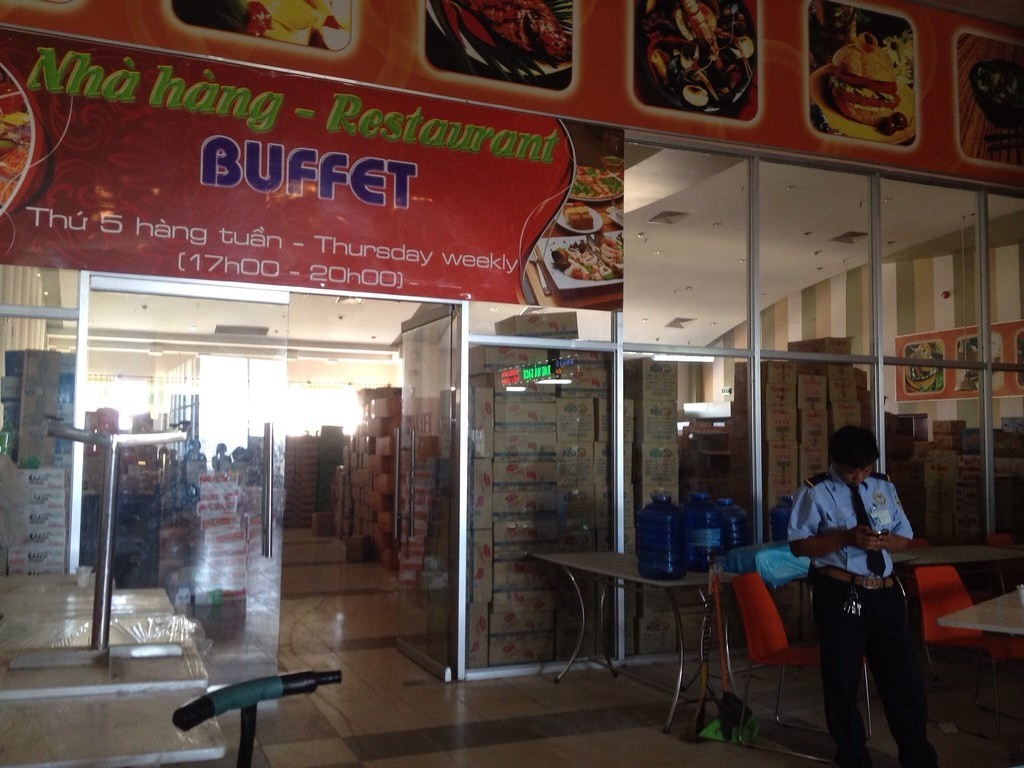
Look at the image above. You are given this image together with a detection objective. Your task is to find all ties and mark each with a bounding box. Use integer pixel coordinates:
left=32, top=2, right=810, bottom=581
left=847, top=484, right=886, bottom=576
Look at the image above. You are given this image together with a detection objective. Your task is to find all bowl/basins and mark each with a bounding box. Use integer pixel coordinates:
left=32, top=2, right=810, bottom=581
left=906, top=365, right=936, bottom=389
left=602, top=157, right=623, bottom=171
left=971, top=58, right=1024, bottom=128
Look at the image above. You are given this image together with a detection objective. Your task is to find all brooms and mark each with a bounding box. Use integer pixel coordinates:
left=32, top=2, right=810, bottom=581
left=710, top=573, right=754, bottom=742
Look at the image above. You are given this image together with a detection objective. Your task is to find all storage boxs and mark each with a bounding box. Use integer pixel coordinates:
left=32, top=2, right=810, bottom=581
left=9, top=309, right=1024, bottom=667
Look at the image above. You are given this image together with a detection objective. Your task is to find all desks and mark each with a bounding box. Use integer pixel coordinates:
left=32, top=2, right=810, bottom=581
left=906, top=545, right=1020, bottom=593
left=937, top=585, right=1024, bottom=635
left=537, top=550, right=753, bottom=738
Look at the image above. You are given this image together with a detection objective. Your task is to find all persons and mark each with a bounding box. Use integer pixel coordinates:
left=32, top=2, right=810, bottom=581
left=787, top=424, right=938, bottom=768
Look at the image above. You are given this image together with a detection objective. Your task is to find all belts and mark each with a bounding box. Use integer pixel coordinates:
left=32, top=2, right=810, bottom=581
left=810, top=562, right=894, bottom=590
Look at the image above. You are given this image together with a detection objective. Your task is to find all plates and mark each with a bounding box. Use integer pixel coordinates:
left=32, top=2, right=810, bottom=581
left=639, top=0, right=757, bottom=111
left=538, top=230, right=623, bottom=290
left=426, top=0, right=573, bottom=76
left=557, top=203, right=602, bottom=233
left=809, top=63, right=915, bottom=144
left=569, top=166, right=624, bottom=202
left=607, top=209, right=623, bottom=227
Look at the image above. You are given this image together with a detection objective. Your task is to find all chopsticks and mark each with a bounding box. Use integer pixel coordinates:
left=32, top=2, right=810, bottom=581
left=985, top=133, right=1024, bottom=151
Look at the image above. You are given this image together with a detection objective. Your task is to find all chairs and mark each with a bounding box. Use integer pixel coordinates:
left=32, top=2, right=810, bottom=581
left=915, top=565, right=1024, bottom=740
left=732, top=570, right=872, bottom=767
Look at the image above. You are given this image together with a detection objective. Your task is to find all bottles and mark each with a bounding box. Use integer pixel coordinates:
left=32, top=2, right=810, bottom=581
left=637, top=494, right=688, bottom=580
left=0, top=421, right=14, bottom=455
left=715, top=498, right=747, bottom=557
left=682, top=493, right=721, bottom=573
left=18, top=455, right=46, bottom=469
left=771, top=495, right=793, bottom=542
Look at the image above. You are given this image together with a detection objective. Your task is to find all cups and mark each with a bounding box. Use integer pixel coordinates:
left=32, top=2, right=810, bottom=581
left=604, top=131, right=623, bottom=158
left=1017, top=584, right=1024, bottom=608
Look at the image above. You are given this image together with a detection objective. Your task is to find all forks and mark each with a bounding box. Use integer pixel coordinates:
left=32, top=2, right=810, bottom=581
left=529, top=250, right=550, bottom=295
